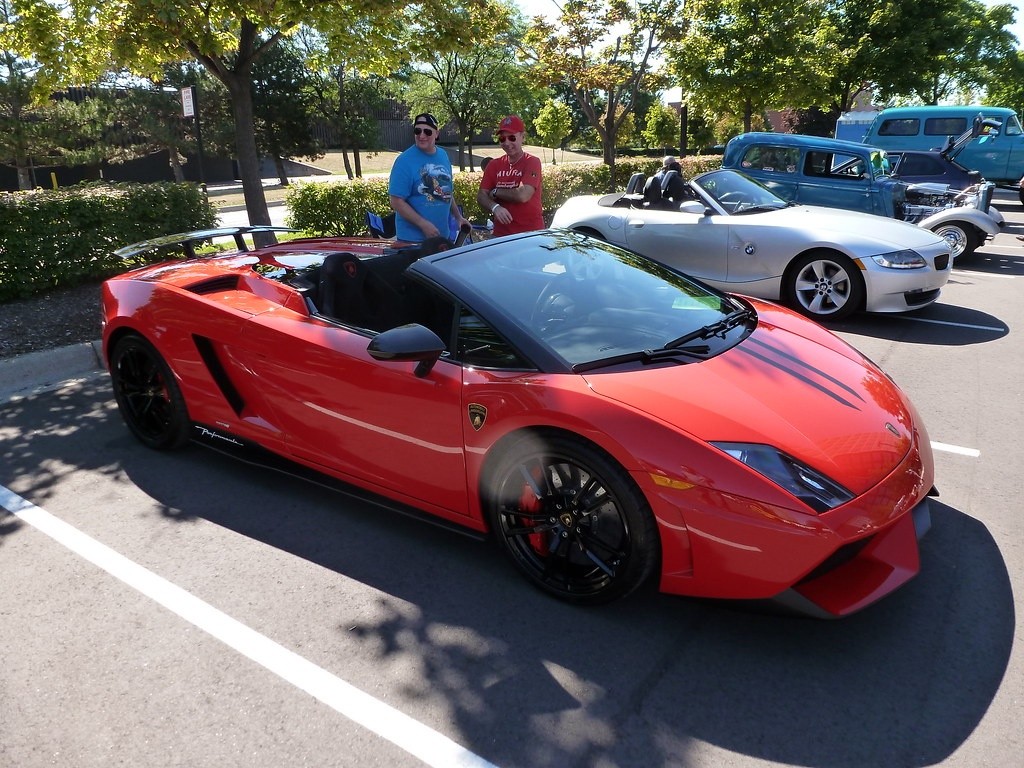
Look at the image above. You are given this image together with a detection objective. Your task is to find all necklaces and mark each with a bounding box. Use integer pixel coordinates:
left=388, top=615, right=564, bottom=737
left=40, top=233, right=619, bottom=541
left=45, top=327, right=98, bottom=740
left=507, top=153, right=524, bottom=169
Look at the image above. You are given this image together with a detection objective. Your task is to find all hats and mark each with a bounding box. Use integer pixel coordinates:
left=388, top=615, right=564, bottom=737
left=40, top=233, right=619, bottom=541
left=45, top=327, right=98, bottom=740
left=497, top=116, right=525, bottom=133
left=661, top=156, right=675, bottom=170
left=413, top=113, right=440, bottom=141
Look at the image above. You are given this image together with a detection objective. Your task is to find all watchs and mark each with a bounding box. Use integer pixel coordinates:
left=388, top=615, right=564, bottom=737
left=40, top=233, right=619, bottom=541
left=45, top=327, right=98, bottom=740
left=492, top=188, right=498, bottom=197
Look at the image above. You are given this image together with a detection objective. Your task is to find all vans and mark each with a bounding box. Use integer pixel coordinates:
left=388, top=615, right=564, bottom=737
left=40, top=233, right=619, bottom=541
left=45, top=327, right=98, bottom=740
left=862, top=106, right=1024, bottom=187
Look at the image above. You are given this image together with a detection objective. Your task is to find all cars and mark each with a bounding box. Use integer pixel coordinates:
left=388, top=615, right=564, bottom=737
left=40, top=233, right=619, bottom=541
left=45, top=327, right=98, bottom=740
left=551, top=169, right=952, bottom=324
left=826, top=129, right=984, bottom=193
left=722, top=132, right=1005, bottom=264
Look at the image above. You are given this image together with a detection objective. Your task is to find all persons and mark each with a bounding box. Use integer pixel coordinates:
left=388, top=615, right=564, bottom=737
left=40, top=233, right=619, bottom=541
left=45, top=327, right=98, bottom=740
left=387, top=112, right=472, bottom=243
left=475, top=116, right=544, bottom=240
left=653, top=156, right=676, bottom=188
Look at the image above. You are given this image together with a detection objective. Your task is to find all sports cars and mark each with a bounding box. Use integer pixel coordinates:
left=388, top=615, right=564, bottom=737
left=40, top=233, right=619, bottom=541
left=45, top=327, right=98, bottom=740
left=102, top=226, right=940, bottom=617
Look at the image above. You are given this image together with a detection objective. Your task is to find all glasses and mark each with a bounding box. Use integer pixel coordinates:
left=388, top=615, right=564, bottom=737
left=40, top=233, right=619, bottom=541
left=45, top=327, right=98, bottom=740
left=414, top=128, right=436, bottom=136
left=499, top=135, right=522, bottom=142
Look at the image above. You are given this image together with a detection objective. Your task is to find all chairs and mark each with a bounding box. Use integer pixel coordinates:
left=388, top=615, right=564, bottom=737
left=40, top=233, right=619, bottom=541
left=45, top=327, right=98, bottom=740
left=317, top=237, right=519, bottom=365
left=643, top=172, right=711, bottom=214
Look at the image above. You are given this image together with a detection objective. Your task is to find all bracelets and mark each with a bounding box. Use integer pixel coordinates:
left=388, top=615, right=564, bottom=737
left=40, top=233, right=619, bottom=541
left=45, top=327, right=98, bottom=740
left=491, top=204, right=501, bottom=213
